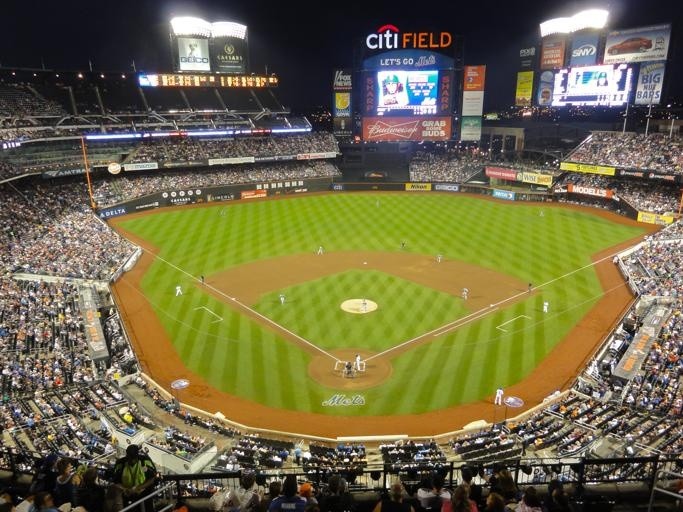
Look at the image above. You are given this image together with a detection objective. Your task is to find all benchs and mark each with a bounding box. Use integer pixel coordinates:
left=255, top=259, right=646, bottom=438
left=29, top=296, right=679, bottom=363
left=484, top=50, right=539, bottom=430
left=0, top=190, right=681, bottom=512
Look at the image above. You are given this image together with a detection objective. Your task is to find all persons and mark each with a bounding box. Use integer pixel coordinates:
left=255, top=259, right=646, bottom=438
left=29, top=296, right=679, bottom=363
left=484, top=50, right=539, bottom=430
left=461, top=287, right=468, bottom=300
left=437, top=254, right=441, bottom=264
left=360, top=296, right=368, bottom=311
left=317, top=242, right=323, bottom=255
left=353, top=352, right=361, bottom=370
left=401, top=239, right=406, bottom=248
left=279, top=292, right=285, bottom=304
left=346, top=362, right=354, bottom=378
left=175, top=286, right=182, bottom=296
left=527, top=282, right=533, bottom=296
left=201, top=274, right=205, bottom=285
left=569, top=214, right=683, bottom=511
left=543, top=298, right=548, bottom=314
